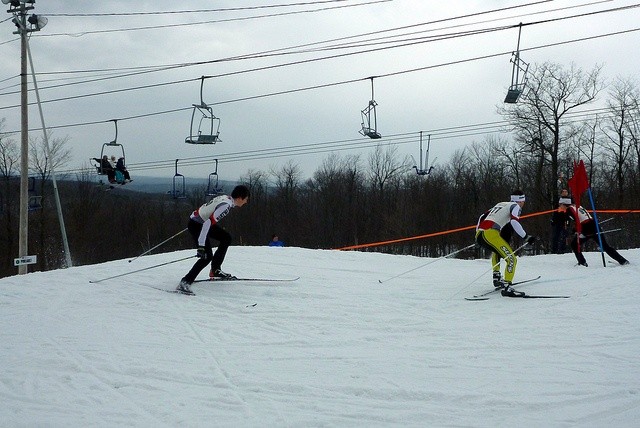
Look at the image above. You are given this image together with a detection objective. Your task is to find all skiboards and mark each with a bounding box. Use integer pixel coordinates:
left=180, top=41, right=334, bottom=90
left=465, top=275, right=571, bottom=300
left=154, top=277, right=300, bottom=307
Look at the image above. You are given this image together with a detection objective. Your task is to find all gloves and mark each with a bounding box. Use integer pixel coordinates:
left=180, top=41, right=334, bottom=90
left=523, top=233, right=535, bottom=245
left=577, top=233, right=585, bottom=239
left=197, top=248, right=207, bottom=259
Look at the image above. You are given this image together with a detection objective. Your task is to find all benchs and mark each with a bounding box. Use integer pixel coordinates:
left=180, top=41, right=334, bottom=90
left=504, top=51, right=530, bottom=104
left=358, top=100, right=382, bottom=139
left=205, top=173, right=227, bottom=196
left=89, top=144, right=126, bottom=176
left=185, top=104, right=222, bottom=145
left=171, top=174, right=188, bottom=199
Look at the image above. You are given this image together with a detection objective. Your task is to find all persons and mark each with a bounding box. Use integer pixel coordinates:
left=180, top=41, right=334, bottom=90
left=558, top=197, right=630, bottom=268
left=267, top=234, right=286, bottom=247
left=474, top=190, right=533, bottom=296
left=116, top=155, right=131, bottom=182
left=91, top=155, right=116, bottom=185
left=108, top=154, right=126, bottom=186
left=175, top=184, right=252, bottom=296
left=556, top=170, right=576, bottom=252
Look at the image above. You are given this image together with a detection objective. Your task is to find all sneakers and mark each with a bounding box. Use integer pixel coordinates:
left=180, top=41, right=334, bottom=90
left=493, top=271, right=504, bottom=287
left=175, top=281, right=193, bottom=295
left=501, top=281, right=525, bottom=297
left=209, top=269, right=231, bottom=279
